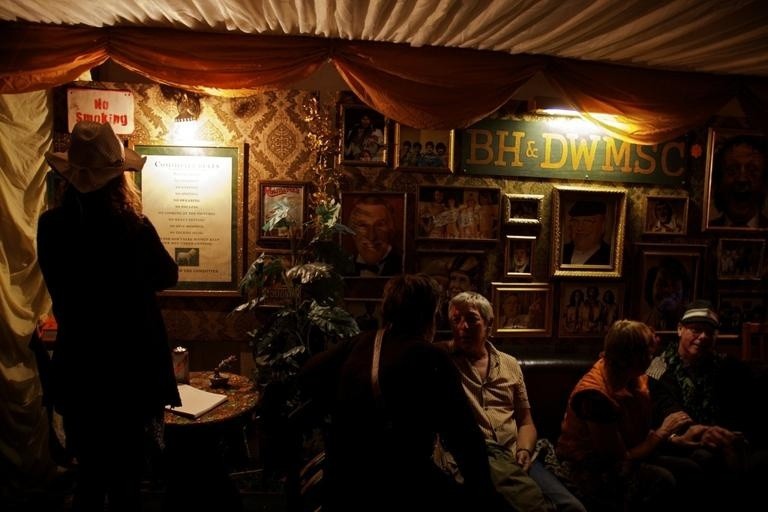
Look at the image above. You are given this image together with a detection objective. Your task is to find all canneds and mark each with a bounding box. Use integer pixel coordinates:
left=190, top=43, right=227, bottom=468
left=172, top=346, right=190, bottom=384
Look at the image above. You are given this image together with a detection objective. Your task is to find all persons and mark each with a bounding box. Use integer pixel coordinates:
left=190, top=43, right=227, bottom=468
left=34, top=119, right=184, bottom=510
left=645, top=305, right=753, bottom=511
left=430, top=292, right=587, bottom=512
left=554, top=319, right=711, bottom=510
left=292, top=272, right=497, bottom=511
left=343, top=116, right=764, bottom=336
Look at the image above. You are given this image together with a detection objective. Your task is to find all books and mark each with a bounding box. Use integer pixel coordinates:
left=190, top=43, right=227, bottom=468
left=164, top=384, right=228, bottom=419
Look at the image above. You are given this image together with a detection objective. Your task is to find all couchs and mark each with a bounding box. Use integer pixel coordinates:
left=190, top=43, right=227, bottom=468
left=289, top=361, right=597, bottom=511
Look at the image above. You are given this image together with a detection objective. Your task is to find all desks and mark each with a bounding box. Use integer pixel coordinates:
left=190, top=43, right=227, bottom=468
left=163, top=370, right=261, bottom=427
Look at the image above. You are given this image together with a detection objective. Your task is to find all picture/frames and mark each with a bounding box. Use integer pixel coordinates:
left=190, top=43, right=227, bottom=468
left=333, top=89, right=399, bottom=170
left=133, top=140, right=245, bottom=298
left=255, top=180, right=309, bottom=246
left=700, top=127, right=767, bottom=233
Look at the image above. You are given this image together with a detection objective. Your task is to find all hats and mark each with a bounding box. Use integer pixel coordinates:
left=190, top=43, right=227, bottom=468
left=678, top=299, right=724, bottom=330
left=42, top=117, right=147, bottom=194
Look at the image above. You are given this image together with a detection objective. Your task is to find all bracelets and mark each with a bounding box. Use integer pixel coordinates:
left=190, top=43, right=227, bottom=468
left=516, top=448, right=532, bottom=457
